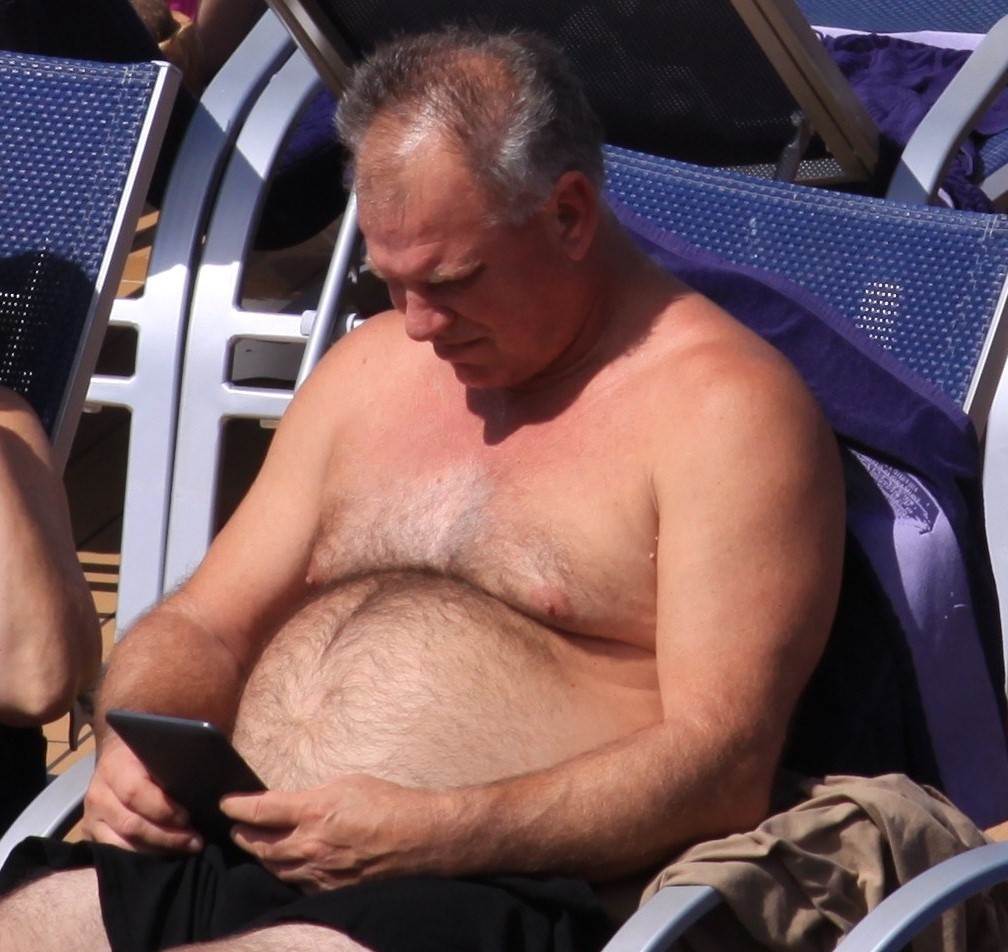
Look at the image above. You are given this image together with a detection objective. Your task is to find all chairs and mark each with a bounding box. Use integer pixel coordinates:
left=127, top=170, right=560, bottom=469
left=0, top=0, right=1008, bottom=952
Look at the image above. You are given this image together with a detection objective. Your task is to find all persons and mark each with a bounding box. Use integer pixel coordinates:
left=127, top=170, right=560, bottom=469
left=0, top=382, right=102, bottom=831
left=0, top=27, right=849, bottom=951
left=131, top=0, right=232, bottom=86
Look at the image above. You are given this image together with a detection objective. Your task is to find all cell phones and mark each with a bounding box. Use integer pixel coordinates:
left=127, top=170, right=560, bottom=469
left=107, top=710, right=271, bottom=839
